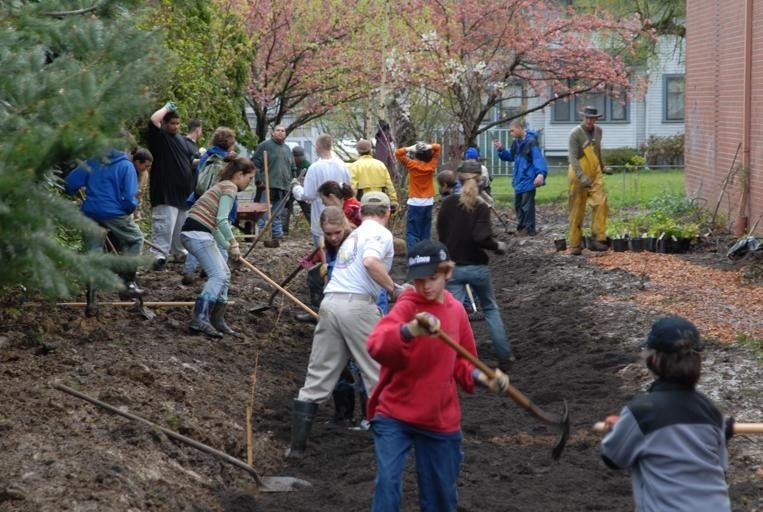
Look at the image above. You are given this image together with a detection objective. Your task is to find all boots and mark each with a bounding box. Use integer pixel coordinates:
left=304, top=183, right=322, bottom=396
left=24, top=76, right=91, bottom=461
left=185, top=296, right=222, bottom=339
left=85, top=280, right=102, bottom=319
left=284, top=397, right=319, bottom=463
left=322, top=369, right=368, bottom=431
left=587, top=237, right=610, bottom=252
left=210, top=302, right=234, bottom=335
left=571, top=245, right=582, bottom=256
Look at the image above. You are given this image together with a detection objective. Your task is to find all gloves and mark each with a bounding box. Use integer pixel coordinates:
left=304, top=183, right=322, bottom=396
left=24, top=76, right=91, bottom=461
left=474, top=368, right=509, bottom=395
left=404, top=312, right=441, bottom=340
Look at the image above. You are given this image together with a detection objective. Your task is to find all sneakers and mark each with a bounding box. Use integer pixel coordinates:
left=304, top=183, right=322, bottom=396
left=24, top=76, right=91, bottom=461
left=120, top=279, right=150, bottom=302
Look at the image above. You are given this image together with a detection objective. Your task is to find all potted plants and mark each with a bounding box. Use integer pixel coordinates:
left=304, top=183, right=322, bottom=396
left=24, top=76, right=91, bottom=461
left=549, top=206, right=704, bottom=256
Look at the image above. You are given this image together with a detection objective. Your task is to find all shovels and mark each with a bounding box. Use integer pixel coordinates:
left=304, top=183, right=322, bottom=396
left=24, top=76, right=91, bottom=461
left=415, top=313, right=570, bottom=459
left=706, top=142, right=742, bottom=232
left=248, top=245, right=320, bottom=312
left=57, top=383, right=313, bottom=493
left=78, top=187, right=156, bottom=321
left=263, top=150, right=280, bottom=248
left=491, top=207, right=514, bottom=234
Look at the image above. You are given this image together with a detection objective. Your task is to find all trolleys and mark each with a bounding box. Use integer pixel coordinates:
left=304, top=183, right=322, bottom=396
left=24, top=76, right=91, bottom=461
left=236, top=201, right=273, bottom=241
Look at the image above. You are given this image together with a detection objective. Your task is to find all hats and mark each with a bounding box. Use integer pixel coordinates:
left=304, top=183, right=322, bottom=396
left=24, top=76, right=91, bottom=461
left=405, top=240, right=450, bottom=279
left=578, top=105, right=604, bottom=118
left=649, top=315, right=700, bottom=350
left=358, top=140, right=372, bottom=153
left=455, top=161, right=483, bottom=176
left=360, top=190, right=390, bottom=209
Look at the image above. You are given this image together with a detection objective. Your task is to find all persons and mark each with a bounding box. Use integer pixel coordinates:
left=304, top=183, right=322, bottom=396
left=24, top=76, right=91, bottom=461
left=602, top=316, right=734, bottom=511
left=567, top=106, right=614, bottom=255
left=492, top=120, right=548, bottom=236
